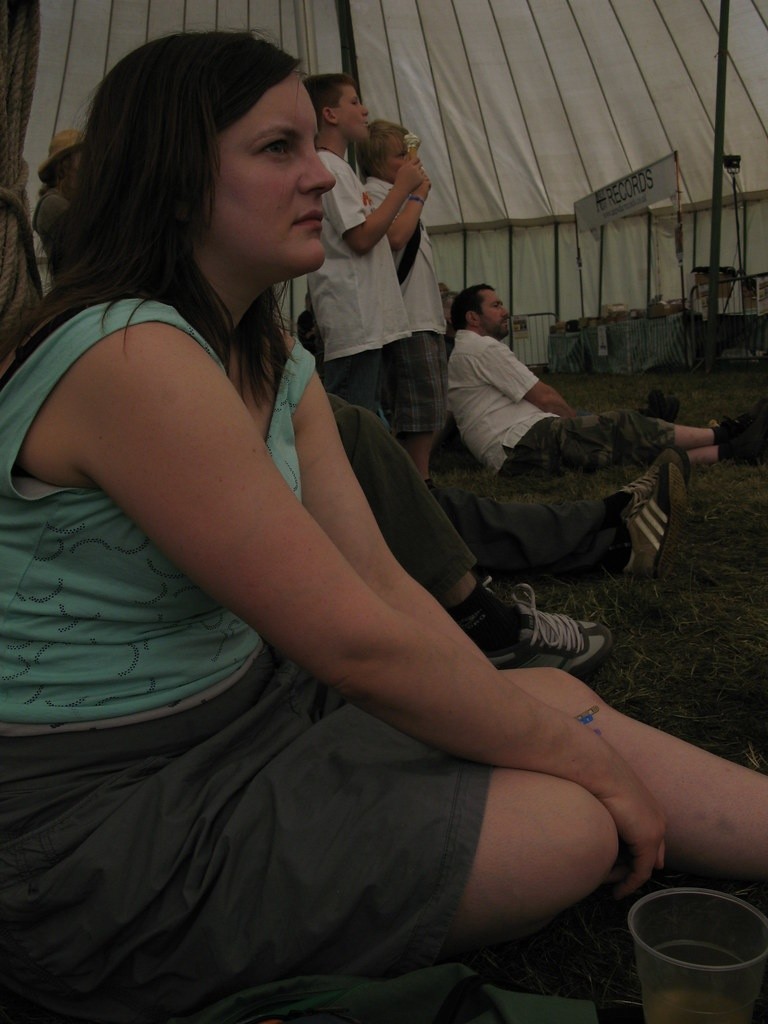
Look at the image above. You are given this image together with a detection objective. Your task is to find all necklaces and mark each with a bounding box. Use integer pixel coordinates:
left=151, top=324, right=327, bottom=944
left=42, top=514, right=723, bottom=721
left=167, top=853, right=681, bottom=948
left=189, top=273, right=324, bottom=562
left=316, top=147, right=348, bottom=164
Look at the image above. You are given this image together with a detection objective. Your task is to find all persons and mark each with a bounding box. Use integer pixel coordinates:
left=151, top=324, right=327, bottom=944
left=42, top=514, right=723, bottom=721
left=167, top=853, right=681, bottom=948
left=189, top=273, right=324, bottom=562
left=0, top=31, right=768, bottom=1022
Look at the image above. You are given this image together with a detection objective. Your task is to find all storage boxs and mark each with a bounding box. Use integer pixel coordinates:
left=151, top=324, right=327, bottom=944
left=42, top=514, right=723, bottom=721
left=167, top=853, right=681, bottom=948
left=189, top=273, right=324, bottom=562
left=650, top=302, right=682, bottom=318
left=694, top=274, right=732, bottom=298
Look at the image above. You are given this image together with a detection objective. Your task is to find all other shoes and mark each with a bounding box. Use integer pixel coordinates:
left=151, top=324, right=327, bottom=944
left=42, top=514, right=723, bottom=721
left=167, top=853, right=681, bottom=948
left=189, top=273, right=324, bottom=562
left=720, top=398, right=768, bottom=466
left=645, top=389, right=680, bottom=423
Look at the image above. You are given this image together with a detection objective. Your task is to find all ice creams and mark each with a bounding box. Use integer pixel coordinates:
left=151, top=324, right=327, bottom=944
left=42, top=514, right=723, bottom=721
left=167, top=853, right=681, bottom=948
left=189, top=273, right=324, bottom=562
left=402, top=131, right=421, bottom=162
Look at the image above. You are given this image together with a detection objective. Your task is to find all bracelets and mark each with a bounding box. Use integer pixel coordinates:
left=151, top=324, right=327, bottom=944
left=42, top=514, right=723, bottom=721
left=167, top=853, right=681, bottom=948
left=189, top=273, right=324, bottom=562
left=408, top=195, right=425, bottom=205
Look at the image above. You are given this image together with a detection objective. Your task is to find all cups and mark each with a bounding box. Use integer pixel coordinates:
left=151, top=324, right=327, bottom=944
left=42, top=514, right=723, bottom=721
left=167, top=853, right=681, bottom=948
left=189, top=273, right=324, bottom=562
left=626, top=887, right=768, bottom=1024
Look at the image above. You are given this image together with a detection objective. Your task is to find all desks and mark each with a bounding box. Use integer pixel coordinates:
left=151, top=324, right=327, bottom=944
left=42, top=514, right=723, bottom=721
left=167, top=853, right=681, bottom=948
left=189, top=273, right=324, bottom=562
left=543, top=310, right=768, bottom=382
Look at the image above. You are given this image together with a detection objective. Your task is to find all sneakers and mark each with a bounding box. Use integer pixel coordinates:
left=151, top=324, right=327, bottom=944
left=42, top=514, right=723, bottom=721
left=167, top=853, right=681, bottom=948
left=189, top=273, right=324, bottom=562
left=484, top=581, right=611, bottom=679
left=616, top=451, right=686, bottom=538
left=622, top=461, right=687, bottom=583
